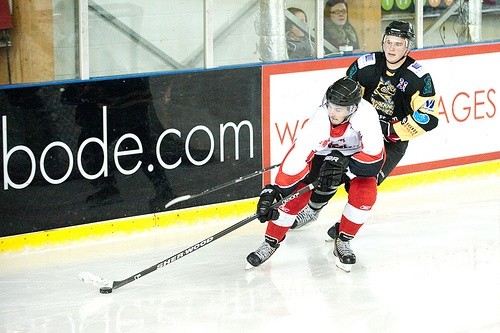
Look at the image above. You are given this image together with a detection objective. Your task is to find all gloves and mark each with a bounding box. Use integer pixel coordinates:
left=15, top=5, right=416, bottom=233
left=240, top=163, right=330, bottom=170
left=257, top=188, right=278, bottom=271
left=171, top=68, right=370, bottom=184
left=318, top=150, right=349, bottom=192
left=257, top=184, right=283, bottom=223
left=380, top=118, right=397, bottom=147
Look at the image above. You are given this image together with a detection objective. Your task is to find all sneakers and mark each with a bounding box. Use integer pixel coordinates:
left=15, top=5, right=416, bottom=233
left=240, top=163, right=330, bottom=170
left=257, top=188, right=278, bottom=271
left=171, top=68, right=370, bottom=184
left=245, top=234, right=286, bottom=269
left=289, top=205, right=322, bottom=231
left=332, top=222, right=356, bottom=273
left=149, top=180, right=181, bottom=213
left=325, top=219, right=341, bottom=243
left=86, top=175, right=122, bottom=207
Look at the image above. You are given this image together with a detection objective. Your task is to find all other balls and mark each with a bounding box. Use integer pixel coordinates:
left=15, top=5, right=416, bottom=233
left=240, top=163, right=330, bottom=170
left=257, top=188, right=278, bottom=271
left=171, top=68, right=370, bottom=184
left=100, top=287, right=112, bottom=294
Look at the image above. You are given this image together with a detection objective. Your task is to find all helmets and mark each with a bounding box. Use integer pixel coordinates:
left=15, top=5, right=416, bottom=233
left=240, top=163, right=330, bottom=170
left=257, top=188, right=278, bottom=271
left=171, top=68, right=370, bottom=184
left=385, top=20, right=418, bottom=43
left=326, top=76, right=362, bottom=107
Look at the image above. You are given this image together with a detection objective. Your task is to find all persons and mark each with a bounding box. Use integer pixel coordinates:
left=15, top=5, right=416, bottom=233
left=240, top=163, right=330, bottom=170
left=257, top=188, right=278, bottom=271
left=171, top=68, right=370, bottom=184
left=289, top=21, right=438, bottom=241
left=284, top=8, right=316, bottom=60
left=244, top=76, right=386, bottom=273
left=324, top=0, right=363, bottom=57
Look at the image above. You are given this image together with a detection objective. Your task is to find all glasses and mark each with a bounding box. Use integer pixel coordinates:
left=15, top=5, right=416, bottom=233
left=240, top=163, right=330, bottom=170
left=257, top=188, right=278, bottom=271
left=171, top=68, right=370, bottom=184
left=329, top=9, right=347, bottom=15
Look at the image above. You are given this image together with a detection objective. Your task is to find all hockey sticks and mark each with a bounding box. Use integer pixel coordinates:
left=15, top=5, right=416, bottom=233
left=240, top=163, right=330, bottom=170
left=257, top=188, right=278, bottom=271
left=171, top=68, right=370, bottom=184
left=78, top=180, right=321, bottom=290
left=165, top=162, right=282, bottom=208
